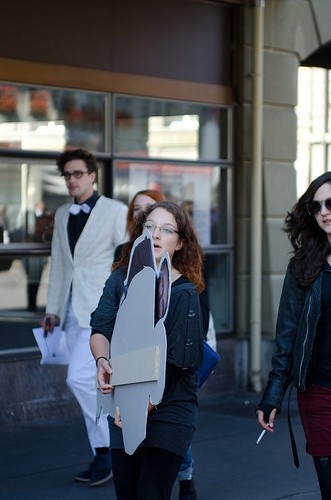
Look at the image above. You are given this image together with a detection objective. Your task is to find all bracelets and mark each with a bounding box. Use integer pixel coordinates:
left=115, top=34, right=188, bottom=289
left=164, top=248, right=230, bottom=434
left=95, top=357, right=109, bottom=367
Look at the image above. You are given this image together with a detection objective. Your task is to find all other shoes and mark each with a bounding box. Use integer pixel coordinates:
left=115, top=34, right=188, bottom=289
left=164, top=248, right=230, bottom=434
left=74, top=449, right=112, bottom=486
left=178, top=480, right=196, bottom=500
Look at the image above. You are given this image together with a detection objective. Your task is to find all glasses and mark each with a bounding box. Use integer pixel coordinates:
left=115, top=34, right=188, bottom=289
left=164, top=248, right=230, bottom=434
left=309, top=198, right=331, bottom=217
left=141, top=223, right=181, bottom=236
left=61, top=170, right=90, bottom=180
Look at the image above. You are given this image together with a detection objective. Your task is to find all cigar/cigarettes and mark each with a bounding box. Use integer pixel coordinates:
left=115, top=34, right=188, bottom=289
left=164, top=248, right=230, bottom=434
left=256, top=422, right=271, bottom=445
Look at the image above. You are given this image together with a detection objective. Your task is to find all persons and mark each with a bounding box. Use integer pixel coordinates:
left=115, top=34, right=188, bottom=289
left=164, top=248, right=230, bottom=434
left=124, top=236, right=170, bottom=328
left=87, top=198, right=207, bottom=499
left=255, top=170, right=331, bottom=499
left=111, top=189, right=199, bottom=500
left=150, top=179, right=222, bottom=230
left=0, top=200, right=55, bottom=313
left=43, top=147, right=133, bottom=487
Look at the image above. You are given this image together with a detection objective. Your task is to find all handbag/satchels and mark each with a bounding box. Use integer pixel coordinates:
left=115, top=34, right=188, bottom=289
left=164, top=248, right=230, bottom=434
left=197, top=341, right=222, bottom=388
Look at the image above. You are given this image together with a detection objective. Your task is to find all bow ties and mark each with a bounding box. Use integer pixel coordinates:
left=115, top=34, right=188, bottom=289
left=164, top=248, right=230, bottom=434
left=68, top=204, right=92, bottom=216
